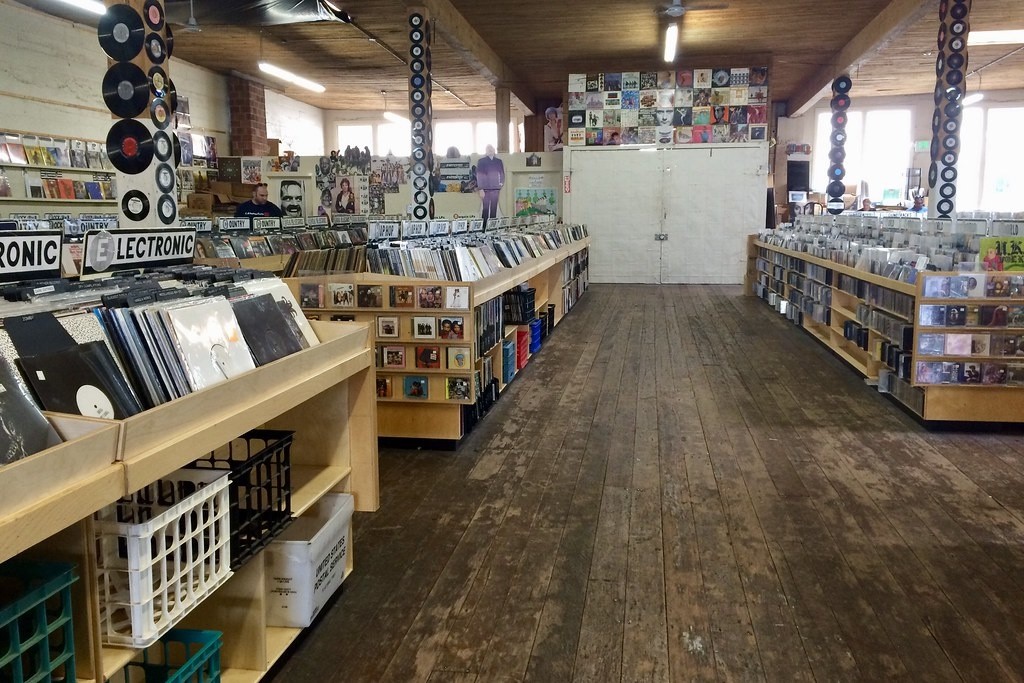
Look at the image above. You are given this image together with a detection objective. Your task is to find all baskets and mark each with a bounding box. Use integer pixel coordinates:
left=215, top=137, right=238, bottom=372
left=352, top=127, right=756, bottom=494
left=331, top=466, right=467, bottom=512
left=502, top=288, right=536, bottom=325
left=106, top=627, right=223, bottom=683
left=95, top=466, right=235, bottom=648
left=539, top=312, right=548, bottom=341
left=112, top=427, right=296, bottom=572
left=548, top=304, right=556, bottom=331
left=504, top=341, right=515, bottom=383
left=530, top=319, right=541, bottom=353
left=0, top=555, right=80, bottom=682
left=517, top=330, right=528, bottom=369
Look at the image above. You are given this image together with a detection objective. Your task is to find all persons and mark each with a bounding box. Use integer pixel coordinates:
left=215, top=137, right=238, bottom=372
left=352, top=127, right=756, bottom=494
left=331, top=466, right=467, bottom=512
left=235, top=184, right=281, bottom=218
left=279, top=68, right=1023, bottom=439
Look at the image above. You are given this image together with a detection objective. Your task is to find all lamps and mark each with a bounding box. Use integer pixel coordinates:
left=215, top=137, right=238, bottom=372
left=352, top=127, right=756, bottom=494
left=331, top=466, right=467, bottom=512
left=963, top=72, right=983, bottom=107
left=260, top=30, right=326, bottom=96
left=382, top=93, right=411, bottom=130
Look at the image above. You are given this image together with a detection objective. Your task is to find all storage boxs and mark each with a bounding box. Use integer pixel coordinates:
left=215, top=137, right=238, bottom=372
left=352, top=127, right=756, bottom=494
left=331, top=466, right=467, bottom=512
left=264, top=492, right=353, bottom=627
left=185, top=183, right=256, bottom=217
left=121, top=430, right=294, bottom=570
left=1, top=550, right=78, bottom=682
left=108, top=627, right=223, bottom=683
left=92, top=468, right=235, bottom=648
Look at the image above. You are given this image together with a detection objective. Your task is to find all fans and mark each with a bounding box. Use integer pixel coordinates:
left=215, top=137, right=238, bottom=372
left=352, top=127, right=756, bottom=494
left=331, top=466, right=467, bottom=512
left=653, top=0, right=729, bottom=17
left=173, top=1, right=203, bottom=36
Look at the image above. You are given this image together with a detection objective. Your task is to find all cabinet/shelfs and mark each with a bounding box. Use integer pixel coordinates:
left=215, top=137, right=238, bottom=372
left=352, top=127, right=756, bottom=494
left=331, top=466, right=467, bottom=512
left=746, top=204, right=1024, bottom=435
left=1, top=127, right=118, bottom=234
left=562, top=141, right=768, bottom=285
left=1, top=412, right=132, bottom=683
left=192, top=228, right=594, bottom=452
left=45, top=320, right=381, bottom=683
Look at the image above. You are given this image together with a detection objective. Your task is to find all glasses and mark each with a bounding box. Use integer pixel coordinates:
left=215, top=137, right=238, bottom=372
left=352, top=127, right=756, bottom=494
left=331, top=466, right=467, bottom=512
left=257, top=183, right=268, bottom=189
